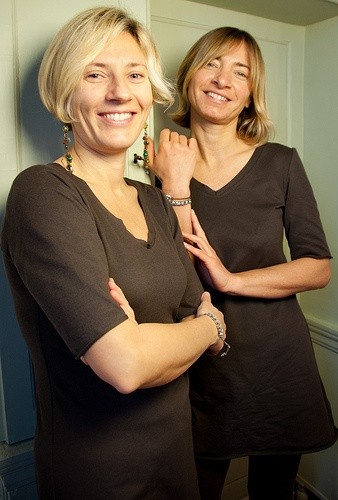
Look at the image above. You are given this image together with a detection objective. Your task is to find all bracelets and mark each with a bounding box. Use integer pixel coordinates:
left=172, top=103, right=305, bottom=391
left=199, top=312, right=230, bottom=357
left=165, top=194, right=191, bottom=206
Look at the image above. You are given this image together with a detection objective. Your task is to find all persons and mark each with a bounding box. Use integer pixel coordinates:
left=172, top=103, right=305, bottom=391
left=145, top=25, right=338, bottom=500
left=0, top=4, right=231, bottom=500
